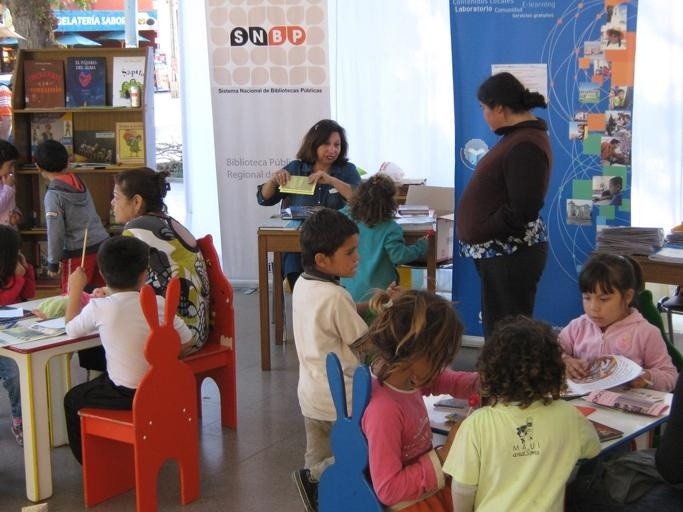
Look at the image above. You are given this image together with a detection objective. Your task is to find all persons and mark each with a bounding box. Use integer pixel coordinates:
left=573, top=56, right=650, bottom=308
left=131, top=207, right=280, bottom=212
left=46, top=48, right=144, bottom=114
left=111, top=168, right=209, bottom=357
left=623, top=255, right=683, bottom=370
left=65, top=121, right=72, bottom=139
left=358, top=286, right=481, bottom=512
left=34, top=140, right=112, bottom=297
left=0, top=223, right=34, bottom=447
left=291, top=208, right=397, bottom=512
left=340, top=175, right=431, bottom=304
left=439, top=317, right=600, bottom=512
left=600, top=139, right=620, bottom=167
left=621, top=116, right=632, bottom=132
left=606, top=27, right=625, bottom=49
left=615, top=89, right=626, bottom=107
left=257, top=121, right=361, bottom=291
left=557, top=253, right=680, bottom=394
left=42, top=122, right=53, bottom=142
left=456, top=72, right=553, bottom=333
left=609, top=176, right=624, bottom=210
left=0, top=139, right=21, bottom=227
left=64, top=236, right=193, bottom=463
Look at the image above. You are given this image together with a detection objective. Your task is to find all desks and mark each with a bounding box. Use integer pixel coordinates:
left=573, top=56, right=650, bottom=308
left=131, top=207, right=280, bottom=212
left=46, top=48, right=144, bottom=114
left=424, top=392, right=674, bottom=462
left=258, top=210, right=437, bottom=371
left=591, top=251, right=682, bottom=286
left=0, top=296, right=101, bottom=501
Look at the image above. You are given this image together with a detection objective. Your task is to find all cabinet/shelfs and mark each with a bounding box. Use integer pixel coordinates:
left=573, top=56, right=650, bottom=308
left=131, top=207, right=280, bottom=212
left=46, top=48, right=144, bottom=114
left=10, top=48, right=155, bottom=298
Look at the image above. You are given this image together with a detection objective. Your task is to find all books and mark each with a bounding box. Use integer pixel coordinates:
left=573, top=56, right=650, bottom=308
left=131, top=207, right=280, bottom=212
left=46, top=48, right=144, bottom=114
left=116, top=120, right=145, bottom=165
left=112, top=57, right=145, bottom=108
left=64, top=57, right=106, bottom=106
left=30, top=113, right=72, bottom=166
left=72, top=131, right=116, bottom=169
left=24, top=58, right=65, bottom=107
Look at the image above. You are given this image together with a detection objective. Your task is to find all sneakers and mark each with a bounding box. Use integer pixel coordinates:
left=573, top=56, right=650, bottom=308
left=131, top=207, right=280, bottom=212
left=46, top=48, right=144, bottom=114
left=292, top=468, right=319, bottom=512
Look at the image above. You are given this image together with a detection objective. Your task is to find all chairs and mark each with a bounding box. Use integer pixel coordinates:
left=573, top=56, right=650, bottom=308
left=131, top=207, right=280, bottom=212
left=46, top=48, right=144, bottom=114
left=656, top=287, right=683, bottom=348
left=403, top=182, right=456, bottom=267
left=176, top=234, right=237, bottom=430
left=79, top=278, right=199, bottom=511
left=316, top=352, right=384, bottom=512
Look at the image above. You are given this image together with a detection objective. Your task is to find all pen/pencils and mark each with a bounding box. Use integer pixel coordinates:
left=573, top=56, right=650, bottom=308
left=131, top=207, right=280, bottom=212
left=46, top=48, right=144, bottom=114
left=80, top=228, right=88, bottom=269
